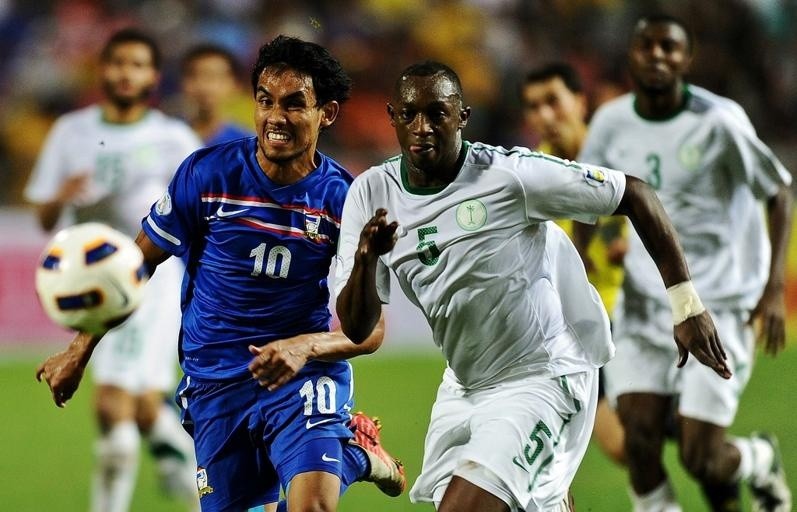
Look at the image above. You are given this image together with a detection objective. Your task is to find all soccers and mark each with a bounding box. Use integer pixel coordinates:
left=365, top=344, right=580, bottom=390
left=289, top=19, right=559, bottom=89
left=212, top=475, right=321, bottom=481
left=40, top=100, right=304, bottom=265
left=36, top=222, right=146, bottom=335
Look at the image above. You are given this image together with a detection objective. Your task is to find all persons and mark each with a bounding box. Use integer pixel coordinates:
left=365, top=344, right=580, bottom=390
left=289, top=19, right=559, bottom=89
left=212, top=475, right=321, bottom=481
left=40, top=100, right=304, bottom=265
left=334, top=59, right=734, bottom=512
left=520, top=12, right=797, bottom=512
left=34, top=35, right=405, bottom=512
left=20, top=29, right=279, bottom=512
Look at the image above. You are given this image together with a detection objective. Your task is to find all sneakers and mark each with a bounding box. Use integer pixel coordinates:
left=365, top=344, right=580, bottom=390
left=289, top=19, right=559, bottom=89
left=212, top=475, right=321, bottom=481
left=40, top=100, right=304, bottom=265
left=348, top=410, right=405, bottom=497
left=747, top=430, right=792, bottom=512
